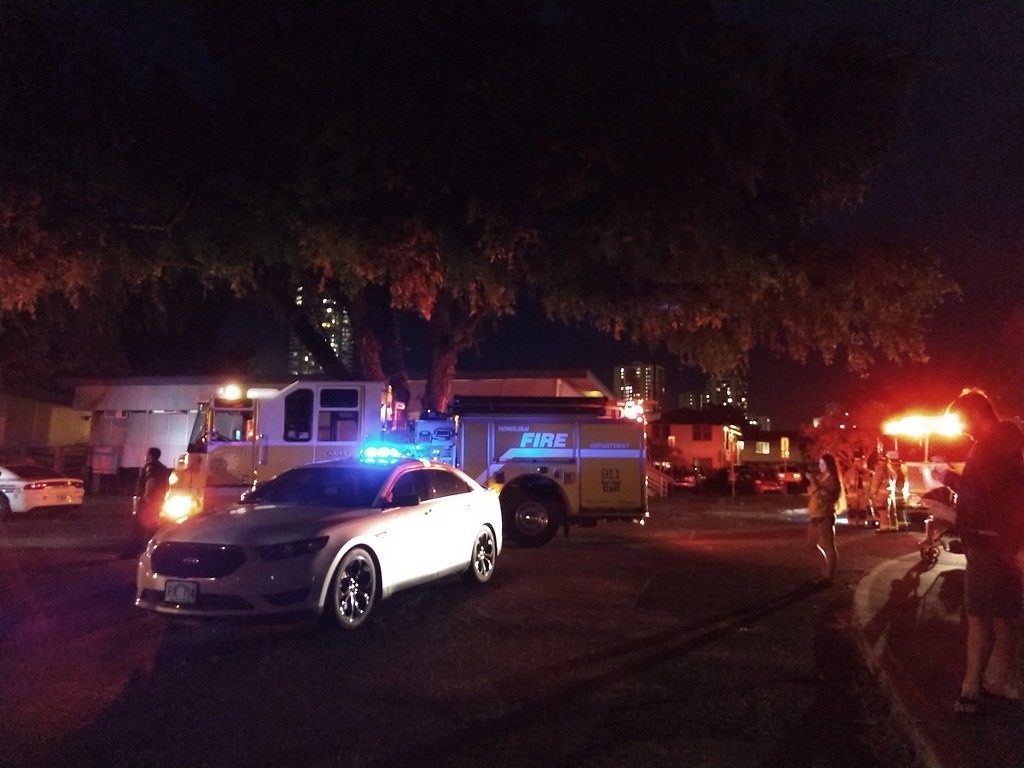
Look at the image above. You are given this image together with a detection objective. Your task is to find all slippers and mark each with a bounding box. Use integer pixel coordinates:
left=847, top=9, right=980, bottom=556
left=953, top=696, right=981, bottom=717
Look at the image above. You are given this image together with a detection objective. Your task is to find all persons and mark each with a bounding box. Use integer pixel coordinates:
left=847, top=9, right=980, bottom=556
left=804, top=386, right=1024, bottom=716
left=117, top=446, right=169, bottom=561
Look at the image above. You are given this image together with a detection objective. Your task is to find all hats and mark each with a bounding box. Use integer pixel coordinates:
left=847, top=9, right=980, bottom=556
left=878, top=456, right=889, bottom=463
left=890, top=458, right=903, bottom=464
left=853, top=454, right=866, bottom=461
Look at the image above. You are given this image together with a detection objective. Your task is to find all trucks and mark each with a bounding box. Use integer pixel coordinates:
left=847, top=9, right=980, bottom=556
left=158, top=376, right=651, bottom=546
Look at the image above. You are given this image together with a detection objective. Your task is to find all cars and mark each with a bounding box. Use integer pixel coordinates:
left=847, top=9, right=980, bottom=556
left=0, top=463, right=85, bottom=521
left=670, top=465, right=706, bottom=490
left=727, top=463, right=802, bottom=496
left=133, top=454, right=503, bottom=634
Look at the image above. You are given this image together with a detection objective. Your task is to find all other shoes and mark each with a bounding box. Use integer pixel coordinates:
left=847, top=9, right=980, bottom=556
left=809, top=575, right=834, bottom=588
